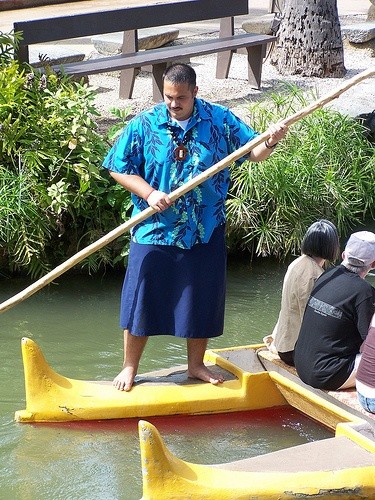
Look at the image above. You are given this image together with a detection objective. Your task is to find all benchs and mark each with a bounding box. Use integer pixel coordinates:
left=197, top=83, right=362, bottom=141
left=14, top=0, right=278, bottom=100
left=255, top=347, right=375, bottom=434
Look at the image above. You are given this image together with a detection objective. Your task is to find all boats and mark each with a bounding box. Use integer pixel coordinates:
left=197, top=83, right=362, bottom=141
left=13, top=336, right=375, bottom=500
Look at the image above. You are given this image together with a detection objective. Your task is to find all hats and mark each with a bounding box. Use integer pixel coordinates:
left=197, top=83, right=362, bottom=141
left=344, top=230, right=375, bottom=267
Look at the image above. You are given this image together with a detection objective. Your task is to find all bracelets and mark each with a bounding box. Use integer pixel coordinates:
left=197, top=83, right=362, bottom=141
left=265, top=139, right=278, bottom=149
left=145, top=188, right=155, bottom=202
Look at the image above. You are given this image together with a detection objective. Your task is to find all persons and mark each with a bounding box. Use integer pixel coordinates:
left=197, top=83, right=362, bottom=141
left=293, top=232, right=375, bottom=390
left=102, top=63, right=289, bottom=391
left=355, top=313, right=375, bottom=414
left=263, top=218, right=339, bottom=367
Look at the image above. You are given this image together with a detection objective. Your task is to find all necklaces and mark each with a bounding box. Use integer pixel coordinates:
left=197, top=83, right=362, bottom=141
left=167, top=111, right=190, bottom=160
